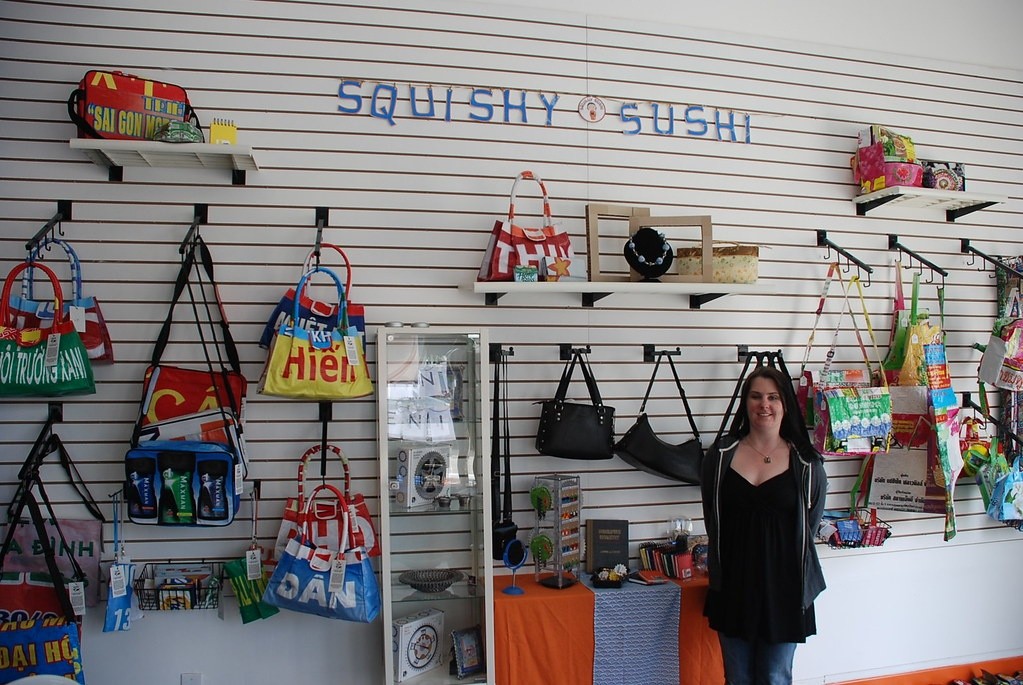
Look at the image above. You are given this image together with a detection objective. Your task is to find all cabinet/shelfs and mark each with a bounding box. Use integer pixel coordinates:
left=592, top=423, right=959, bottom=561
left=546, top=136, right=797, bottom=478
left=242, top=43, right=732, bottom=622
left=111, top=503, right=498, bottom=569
left=375, top=327, right=496, bottom=685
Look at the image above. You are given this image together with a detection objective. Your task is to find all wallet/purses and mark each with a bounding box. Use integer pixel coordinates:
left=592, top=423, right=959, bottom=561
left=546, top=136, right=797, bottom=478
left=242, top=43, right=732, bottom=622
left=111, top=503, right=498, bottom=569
left=99, top=556, right=146, bottom=633
left=513, top=265, right=538, bottom=282
left=817, top=510, right=887, bottom=547
left=153, top=564, right=220, bottom=610
left=542, top=256, right=587, bottom=282
left=223, top=556, right=280, bottom=626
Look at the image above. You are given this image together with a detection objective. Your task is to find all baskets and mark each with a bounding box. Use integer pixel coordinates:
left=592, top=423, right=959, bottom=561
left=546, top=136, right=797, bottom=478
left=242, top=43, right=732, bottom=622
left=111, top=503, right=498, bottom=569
left=818, top=508, right=893, bottom=549
left=135, top=563, right=223, bottom=610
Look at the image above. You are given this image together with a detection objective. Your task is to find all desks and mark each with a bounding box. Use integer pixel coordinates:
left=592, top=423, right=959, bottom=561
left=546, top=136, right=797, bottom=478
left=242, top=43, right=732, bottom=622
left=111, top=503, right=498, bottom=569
left=492, top=568, right=726, bottom=684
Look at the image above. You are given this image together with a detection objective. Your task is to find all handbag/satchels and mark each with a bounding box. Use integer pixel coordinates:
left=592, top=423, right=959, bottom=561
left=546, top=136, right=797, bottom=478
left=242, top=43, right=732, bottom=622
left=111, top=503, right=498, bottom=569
left=256, top=267, right=374, bottom=400
left=676, top=241, right=772, bottom=284
left=0, top=432, right=104, bottom=685
left=959, top=416, right=1023, bottom=521
left=813, top=386, right=893, bottom=456
left=0, top=262, right=97, bottom=398
left=874, top=260, right=950, bottom=388
left=615, top=350, right=705, bottom=486
left=273, top=444, right=381, bottom=561
left=476, top=171, right=576, bottom=282
left=8, top=238, right=114, bottom=365
left=535, top=348, right=616, bottom=460
left=797, top=368, right=883, bottom=432
left=921, top=160, right=966, bottom=191
left=262, top=484, right=381, bottom=623
left=259, top=243, right=367, bottom=365
left=67, top=70, right=206, bottom=143
left=123, top=234, right=249, bottom=527
left=492, top=522, right=518, bottom=561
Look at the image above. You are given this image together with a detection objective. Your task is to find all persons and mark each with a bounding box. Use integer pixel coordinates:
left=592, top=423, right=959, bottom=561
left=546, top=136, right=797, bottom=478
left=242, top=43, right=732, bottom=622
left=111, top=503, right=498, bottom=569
left=700, top=366, right=827, bottom=685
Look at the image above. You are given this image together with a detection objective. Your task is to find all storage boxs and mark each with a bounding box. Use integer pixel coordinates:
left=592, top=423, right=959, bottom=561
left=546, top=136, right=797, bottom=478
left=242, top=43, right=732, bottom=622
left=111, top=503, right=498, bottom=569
left=923, top=163, right=966, bottom=192
left=390, top=608, right=446, bottom=682
left=678, top=246, right=760, bottom=284
left=398, top=443, right=452, bottom=508
left=155, top=564, right=213, bottom=610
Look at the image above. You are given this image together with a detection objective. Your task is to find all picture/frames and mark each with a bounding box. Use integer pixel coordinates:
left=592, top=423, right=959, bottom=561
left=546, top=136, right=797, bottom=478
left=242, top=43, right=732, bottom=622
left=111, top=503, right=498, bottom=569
left=451, top=628, right=483, bottom=679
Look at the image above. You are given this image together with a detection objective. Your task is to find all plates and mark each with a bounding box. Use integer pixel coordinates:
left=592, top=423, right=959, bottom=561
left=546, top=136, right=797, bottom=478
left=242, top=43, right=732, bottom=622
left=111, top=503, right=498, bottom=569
left=399, top=569, right=463, bottom=592
left=590, top=568, right=630, bottom=588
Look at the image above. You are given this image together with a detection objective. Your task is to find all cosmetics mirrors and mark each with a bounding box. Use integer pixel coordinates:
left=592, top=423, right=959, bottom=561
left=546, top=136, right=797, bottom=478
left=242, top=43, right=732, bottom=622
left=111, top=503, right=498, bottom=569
left=502, top=539, right=525, bottom=595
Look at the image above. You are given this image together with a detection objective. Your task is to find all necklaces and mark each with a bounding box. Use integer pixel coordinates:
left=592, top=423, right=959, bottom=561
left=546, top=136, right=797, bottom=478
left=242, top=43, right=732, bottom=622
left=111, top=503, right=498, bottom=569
left=629, top=232, right=669, bottom=266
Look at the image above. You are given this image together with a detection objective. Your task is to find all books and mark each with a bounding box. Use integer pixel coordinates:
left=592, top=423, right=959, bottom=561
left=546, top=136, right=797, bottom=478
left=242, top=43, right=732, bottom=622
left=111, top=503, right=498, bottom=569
left=629, top=541, right=695, bottom=586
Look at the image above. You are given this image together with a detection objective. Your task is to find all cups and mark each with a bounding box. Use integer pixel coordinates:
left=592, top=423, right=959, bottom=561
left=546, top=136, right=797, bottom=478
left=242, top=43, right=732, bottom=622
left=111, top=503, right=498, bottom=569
left=457, top=495, right=471, bottom=505
left=438, top=497, right=451, bottom=507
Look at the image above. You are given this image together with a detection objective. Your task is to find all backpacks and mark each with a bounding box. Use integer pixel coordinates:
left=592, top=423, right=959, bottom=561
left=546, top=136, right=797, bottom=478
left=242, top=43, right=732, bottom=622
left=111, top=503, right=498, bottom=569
left=978, top=288, right=1023, bottom=392
